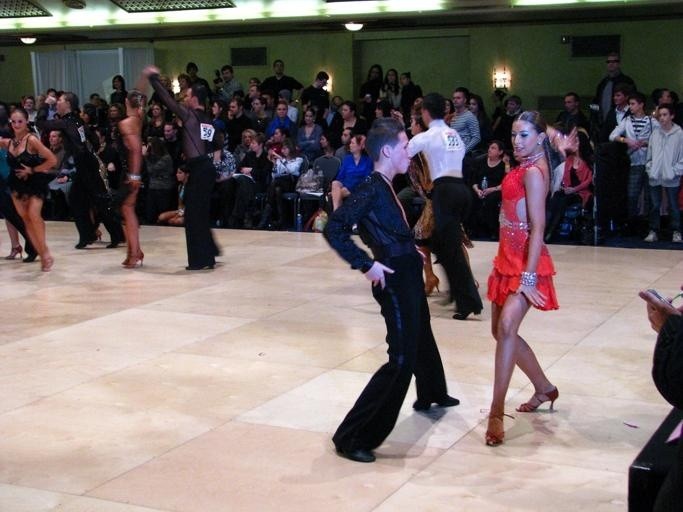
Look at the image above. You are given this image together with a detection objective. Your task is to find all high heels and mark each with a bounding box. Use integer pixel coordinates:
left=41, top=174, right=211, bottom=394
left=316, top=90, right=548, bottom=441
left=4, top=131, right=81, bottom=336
left=485, top=413, right=503, bottom=447
left=424, top=275, right=439, bottom=295
left=5, top=245, right=53, bottom=271
left=453, top=309, right=480, bottom=319
left=122, top=251, right=143, bottom=269
left=515, top=387, right=558, bottom=411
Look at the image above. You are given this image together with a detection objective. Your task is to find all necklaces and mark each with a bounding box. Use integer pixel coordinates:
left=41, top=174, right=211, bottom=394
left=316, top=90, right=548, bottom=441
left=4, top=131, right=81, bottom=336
left=519, top=150, right=547, bottom=166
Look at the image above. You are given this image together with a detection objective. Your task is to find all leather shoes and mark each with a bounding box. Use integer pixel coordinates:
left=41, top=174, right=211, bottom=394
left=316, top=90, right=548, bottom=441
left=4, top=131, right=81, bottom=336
left=434, top=395, right=458, bottom=406
left=334, top=445, right=374, bottom=462
left=75, top=229, right=126, bottom=248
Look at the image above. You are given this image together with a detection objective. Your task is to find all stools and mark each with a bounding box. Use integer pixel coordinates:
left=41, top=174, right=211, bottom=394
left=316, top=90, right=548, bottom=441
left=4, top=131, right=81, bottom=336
left=560, top=203, right=590, bottom=244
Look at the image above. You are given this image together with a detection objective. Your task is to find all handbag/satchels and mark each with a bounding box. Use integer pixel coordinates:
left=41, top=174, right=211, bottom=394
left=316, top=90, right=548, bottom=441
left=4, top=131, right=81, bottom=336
left=311, top=208, right=328, bottom=232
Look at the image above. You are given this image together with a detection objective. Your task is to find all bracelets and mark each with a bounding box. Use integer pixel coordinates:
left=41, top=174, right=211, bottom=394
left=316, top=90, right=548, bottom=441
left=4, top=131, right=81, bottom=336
left=519, top=271, right=538, bottom=286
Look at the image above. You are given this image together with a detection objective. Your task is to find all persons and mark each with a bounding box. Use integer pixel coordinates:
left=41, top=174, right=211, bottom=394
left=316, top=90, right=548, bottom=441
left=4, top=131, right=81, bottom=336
left=0, top=49, right=681, bottom=274
left=626, top=282, right=682, bottom=511
left=480, top=107, right=560, bottom=448
left=320, top=115, right=461, bottom=463
left=405, top=92, right=483, bottom=321
left=406, top=113, right=479, bottom=297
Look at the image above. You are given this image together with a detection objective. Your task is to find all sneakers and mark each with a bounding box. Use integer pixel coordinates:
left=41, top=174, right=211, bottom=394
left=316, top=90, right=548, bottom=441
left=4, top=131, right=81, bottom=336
left=671, top=231, right=681, bottom=242
left=643, top=230, right=657, bottom=242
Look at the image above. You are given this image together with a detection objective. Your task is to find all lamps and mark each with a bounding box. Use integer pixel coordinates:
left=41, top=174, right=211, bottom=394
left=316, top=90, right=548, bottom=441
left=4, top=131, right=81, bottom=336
left=494, top=66, right=512, bottom=94
left=341, top=21, right=364, bottom=31
left=17, top=34, right=38, bottom=45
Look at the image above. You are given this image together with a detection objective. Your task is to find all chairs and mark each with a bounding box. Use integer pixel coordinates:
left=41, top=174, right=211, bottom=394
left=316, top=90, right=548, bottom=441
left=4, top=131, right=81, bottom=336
left=296, top=155, right=340, bottom=226
left=268, top=152, right=310, bottom=224
